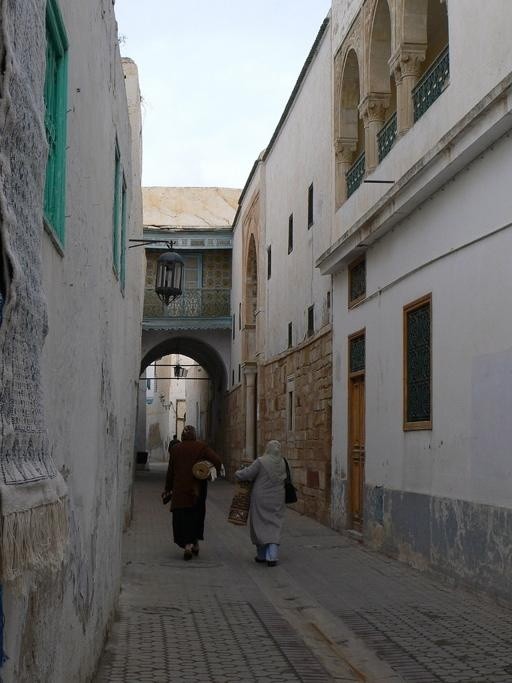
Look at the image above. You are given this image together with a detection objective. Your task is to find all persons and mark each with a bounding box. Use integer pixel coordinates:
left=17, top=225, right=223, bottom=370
left=233, top=442, right=292, bottom=567
left=168, top=434, right=182, bottom=454
left=161, top=425, right=223, bottom=561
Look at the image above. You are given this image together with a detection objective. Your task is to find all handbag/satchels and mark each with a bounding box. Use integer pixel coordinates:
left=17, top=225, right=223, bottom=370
left=283, top=478, right=298, bottom=504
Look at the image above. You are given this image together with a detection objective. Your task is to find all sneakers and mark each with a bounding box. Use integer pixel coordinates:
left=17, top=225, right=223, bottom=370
left=254, top=556, right=276, bottom=567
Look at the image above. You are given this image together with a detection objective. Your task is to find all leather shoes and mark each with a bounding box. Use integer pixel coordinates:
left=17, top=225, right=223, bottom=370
left=184, top=547, right=199, bottom=561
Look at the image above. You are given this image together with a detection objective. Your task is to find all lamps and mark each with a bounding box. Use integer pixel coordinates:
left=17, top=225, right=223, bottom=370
left=129, top=238, right=184, bottom=307
left=173, top=365, right=181, bottom=376
